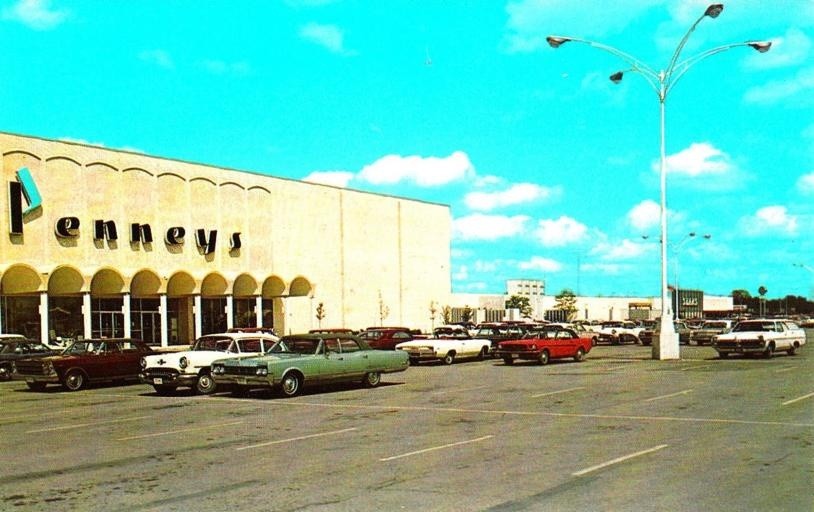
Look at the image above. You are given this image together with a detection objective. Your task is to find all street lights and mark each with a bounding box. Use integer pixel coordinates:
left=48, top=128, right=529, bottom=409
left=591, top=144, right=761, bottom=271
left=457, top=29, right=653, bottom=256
left=641, top=230, right=712, bottom=321
left=546, top=3, right=774, bottom=362
